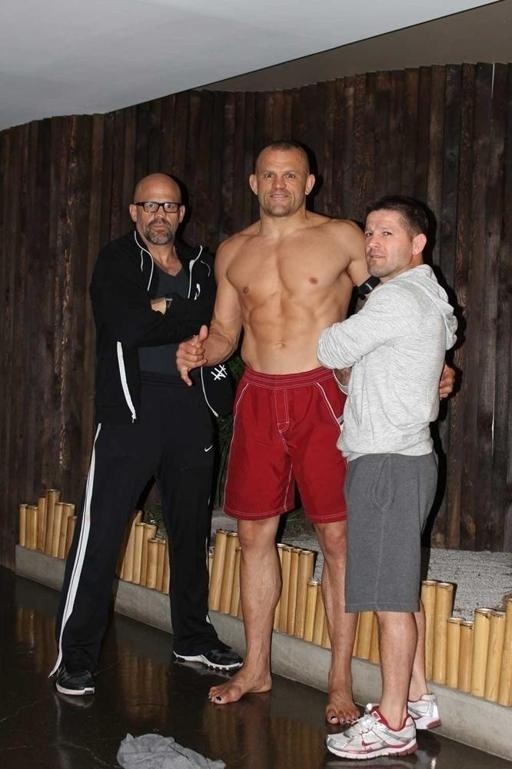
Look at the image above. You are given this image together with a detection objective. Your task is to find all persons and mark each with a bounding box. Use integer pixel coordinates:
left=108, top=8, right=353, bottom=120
left=174, top=138, right=457, bottom=725
left=42, top=168, right=241, bottom=697
left=313, top=195, right=463, bottom=758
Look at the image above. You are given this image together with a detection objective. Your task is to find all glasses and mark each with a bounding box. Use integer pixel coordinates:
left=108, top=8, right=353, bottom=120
left=134, top=200, right=183, bottom=212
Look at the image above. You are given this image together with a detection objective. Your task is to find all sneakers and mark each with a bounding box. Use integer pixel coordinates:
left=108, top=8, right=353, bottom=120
left=365, top=693, right=443, bottom=730
left=322, top=709, right=419, bottom=761
left=54, top=661, right=98, bottom=697
left=172, top=643, right=242, bottom=671
